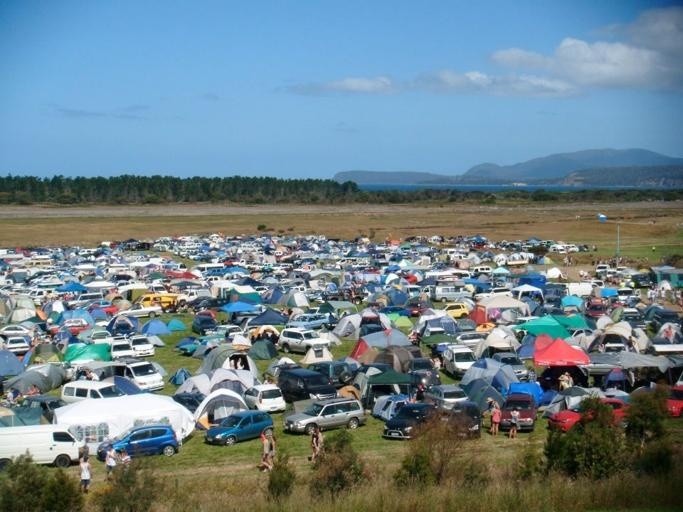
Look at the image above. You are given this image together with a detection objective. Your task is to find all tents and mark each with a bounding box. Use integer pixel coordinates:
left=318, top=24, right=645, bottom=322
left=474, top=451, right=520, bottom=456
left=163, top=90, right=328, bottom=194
left=0, top=232, right=683, bottom=455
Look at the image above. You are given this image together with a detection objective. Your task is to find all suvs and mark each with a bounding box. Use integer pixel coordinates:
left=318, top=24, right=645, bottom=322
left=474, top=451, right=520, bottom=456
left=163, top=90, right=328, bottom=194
left=652, top=308, right=680, bottom=333
left=620, top=307, right=647, bottom=334
left=277, top=326, right=329, bottom=355
left=287, top=396, right=365, bottom=434
left=310, top=360, right=353, bottom=384
left=279, top=368, right=336, bottom=402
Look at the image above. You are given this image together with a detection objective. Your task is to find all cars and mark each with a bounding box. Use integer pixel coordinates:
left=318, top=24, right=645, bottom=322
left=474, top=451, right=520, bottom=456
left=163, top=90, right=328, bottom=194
left=203, top=410, right=274, bottom=446
left=88, top=329, right=113, bottom=346
left=547, top=398, right=632, bottom=434
left=207, top=325, right=244, bottom=339
left=284, top=314, right=328, bottom=330
left=402, top=358, right=442, bottom=386
left=97, top=425, right=178, bottom=461
left=563, top=328, right=595, bottom=343
left=603, top=342, right=626, bottom=354
left=179, top=335, right=228, bottom=357
left=128, top=335, right=156, bottom=358
left=0, top=324, right=42, bottom=338
left=425, top=328, right=445, bottom=337
left=192, top=315, right=217, bottom=334
left=50, top=319, right=89, bottom=334
left=1, top=337, right=31, bottom=355
left=122, top=360, right=164, bottom=394
left=242, top=384, right=287, bottom=414
left=110, top=341, right=136, bottom=363
left=501, top=393, right=539, bottom=431
left=115, top=321, right=135, bottom=336
left=353, top=362, right=395, bottom=378
left=382, top=403, right=441, bottom=441
left=20, top=395, right=68, bottom=422
left=661, top=386, right=682, bottom=418
left=491, top=352, right=529, bottom=382
left=429, top=384, right=472, bottom=412
left=446, top=401, right=482, bottom=438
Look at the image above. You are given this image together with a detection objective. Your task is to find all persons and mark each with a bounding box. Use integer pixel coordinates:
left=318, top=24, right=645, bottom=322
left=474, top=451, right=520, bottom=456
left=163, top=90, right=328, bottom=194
left=78, top=445, right=132, bottom=493
left=260, top=425, right=324, bottom=473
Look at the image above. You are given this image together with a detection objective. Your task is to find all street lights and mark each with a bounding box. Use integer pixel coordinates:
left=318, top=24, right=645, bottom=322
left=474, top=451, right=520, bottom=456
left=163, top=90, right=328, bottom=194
left=616, top=221, right=621, bottom=251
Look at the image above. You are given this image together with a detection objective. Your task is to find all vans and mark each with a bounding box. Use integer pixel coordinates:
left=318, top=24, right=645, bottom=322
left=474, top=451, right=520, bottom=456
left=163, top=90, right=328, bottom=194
left=61, top=380, right=127, bottom=403
left=0, top=423, right=88, bottom=469
left=453, top=330, right=491, bottom=346
left=442, top=345, right=480, bottom=378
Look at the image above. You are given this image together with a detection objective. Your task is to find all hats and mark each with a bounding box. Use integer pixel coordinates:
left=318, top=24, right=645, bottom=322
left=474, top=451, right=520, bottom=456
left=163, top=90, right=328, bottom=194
left=559, top=375, right=566, bottom=380
